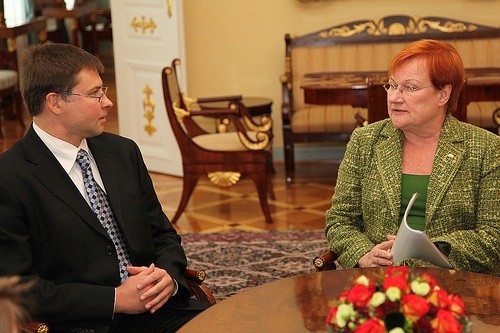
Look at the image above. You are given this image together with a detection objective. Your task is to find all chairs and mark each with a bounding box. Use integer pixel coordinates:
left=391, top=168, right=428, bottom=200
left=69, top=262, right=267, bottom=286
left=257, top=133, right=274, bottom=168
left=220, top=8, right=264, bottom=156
left=162, top=59, right=276, bottom=224
left=313, top=80, right=467, bottom=272
left=0, top=48, right=25, bottom=137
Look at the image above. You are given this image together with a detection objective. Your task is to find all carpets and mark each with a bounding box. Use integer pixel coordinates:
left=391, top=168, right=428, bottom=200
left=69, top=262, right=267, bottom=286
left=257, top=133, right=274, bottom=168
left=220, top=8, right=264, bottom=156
left=180, top=229, right=342, bottom=303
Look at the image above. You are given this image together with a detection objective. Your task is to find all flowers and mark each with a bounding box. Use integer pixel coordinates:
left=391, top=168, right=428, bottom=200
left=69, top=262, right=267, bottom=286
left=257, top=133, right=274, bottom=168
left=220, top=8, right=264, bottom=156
left=324, top=264, right=473, bottom=333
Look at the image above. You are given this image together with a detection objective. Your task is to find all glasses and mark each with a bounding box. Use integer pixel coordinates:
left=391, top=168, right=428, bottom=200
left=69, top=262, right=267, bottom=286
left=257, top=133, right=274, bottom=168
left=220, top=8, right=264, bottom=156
left=68, top=86, right=108, bottom=103
left=383, top=82, right=430, bottom=95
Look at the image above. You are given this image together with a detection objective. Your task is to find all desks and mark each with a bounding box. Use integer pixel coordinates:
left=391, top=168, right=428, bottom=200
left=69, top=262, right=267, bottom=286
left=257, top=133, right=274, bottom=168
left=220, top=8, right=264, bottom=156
left=176, top=266, right=500, bottom=333
left=0, top=3, right=98, bottom=119
left=200, top=97, right=276, bottom=174
left=300, top=73, right=500, bottom=105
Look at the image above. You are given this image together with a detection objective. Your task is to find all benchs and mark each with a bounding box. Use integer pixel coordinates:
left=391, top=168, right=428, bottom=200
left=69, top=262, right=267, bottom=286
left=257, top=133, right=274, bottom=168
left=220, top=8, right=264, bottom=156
left=280, top=15, right=500, bottom=184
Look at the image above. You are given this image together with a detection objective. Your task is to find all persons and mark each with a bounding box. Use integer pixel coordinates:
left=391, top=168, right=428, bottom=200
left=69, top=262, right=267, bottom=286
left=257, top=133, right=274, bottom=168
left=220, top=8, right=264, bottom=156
left=323, top=39, right=500, bottom=280
left=0, top=275, right=38, bottom=333
left=0, top=42, right=211, bottom=333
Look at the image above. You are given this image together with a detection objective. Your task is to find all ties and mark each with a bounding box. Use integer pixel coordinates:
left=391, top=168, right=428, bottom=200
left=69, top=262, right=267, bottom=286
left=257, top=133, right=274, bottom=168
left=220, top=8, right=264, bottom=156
left=75, top=149, right=137, bottom=285
